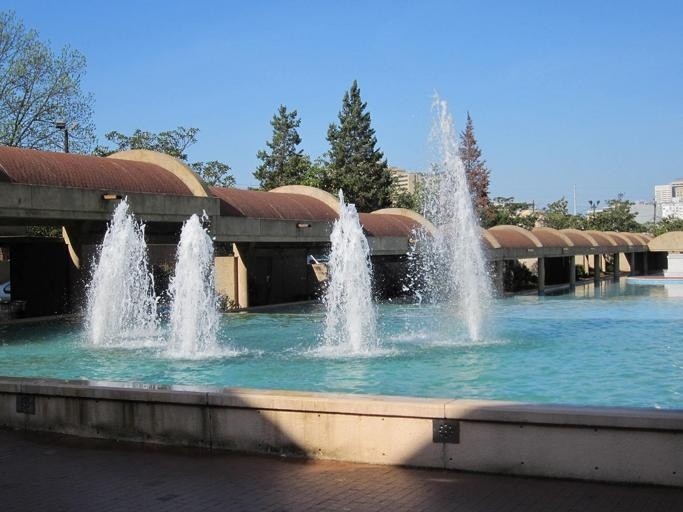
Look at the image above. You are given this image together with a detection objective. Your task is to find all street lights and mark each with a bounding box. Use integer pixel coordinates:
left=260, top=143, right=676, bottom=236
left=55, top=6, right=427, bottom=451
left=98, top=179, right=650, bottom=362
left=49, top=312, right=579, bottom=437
left=588, top=200, right=600, bottom=212
left=54, top=120, right=79, bottom=153
